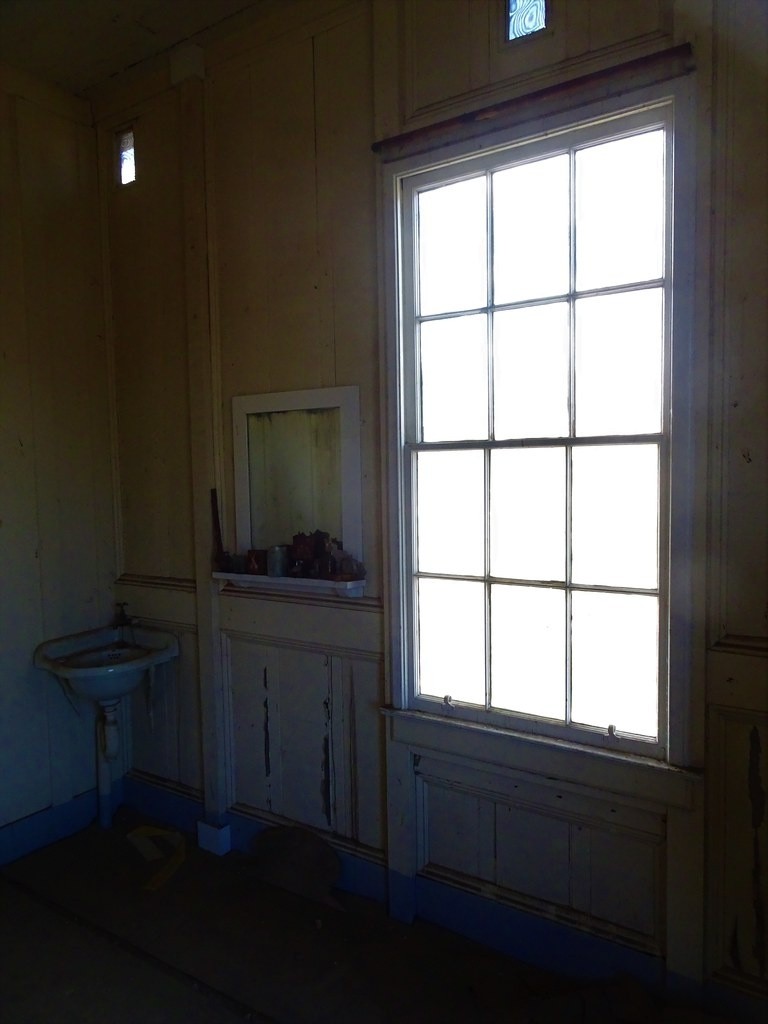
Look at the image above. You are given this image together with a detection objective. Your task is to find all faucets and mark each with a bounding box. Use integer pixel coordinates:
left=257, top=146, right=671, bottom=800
left=112, top=600, right=128, bottom=630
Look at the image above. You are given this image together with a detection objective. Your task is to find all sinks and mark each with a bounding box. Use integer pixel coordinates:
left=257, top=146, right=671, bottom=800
left=33, top=623, right=180, bottom=700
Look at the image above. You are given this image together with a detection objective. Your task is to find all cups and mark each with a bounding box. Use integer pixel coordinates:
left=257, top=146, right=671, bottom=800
left=267, top=545, right=298, bottom=577
left=245, top=550, right=267, bottom=575
